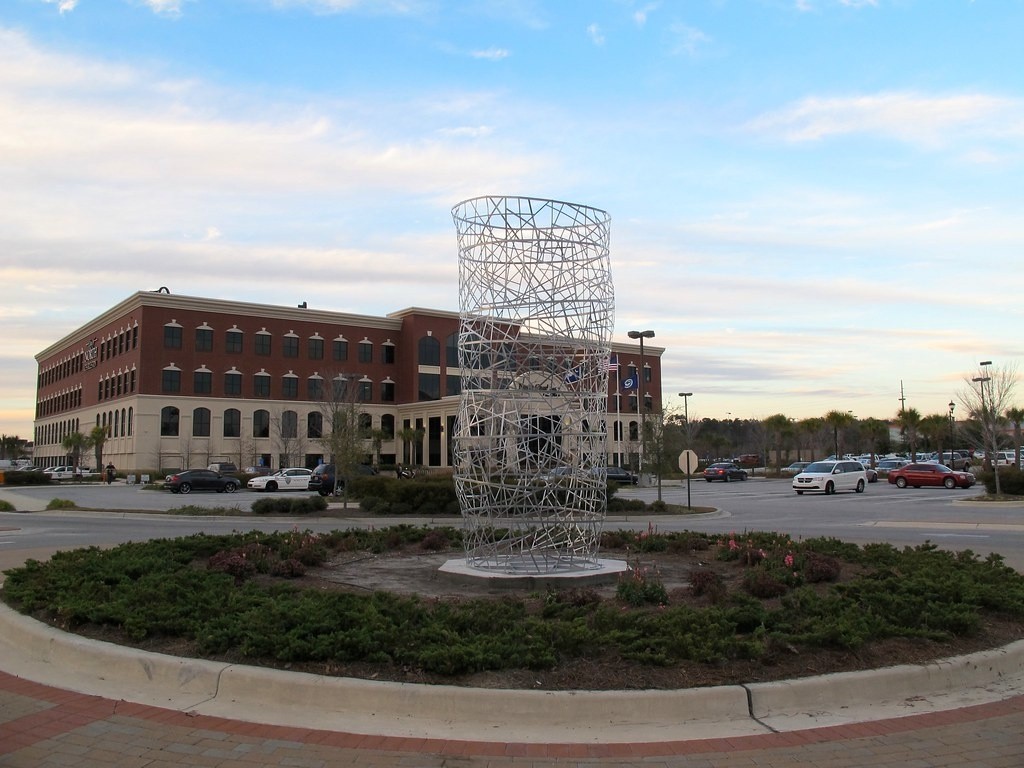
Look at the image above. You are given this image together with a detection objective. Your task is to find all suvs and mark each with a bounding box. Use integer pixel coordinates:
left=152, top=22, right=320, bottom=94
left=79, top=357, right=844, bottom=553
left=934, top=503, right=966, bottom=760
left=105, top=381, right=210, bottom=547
left=207, top=461, right=244, bottom=484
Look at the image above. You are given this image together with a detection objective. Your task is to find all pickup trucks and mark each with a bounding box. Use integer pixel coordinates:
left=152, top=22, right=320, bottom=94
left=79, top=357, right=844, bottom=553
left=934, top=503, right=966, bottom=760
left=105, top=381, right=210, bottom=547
left=926, top=452, right=972, bottom=472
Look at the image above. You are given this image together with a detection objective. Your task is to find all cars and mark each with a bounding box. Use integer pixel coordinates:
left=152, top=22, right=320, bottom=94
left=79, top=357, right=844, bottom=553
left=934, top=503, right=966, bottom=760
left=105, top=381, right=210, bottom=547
left=42, top=467, right=55, bottom=472
left=826, top=446, right=1024, bottom=482
left=584, top=467, right=639, bottom=486
left=163, top=468, right=241, bottom=494
left=704, top=462, right=748, bottom=482
left=780, top=462, right=811, bottom=475
left=43, top=465, right=90, bottom=482
left=792, top=461, right=867, bottom=495
left=888, top=462, right=976, bottom=490
left=864, top=466, right=878, bottom=483
left=247, top=467, right=313, bottom=492
left=31, top=469, right=46, bottom=472
left=245, top=466, right=274, bottom=476
left=308, top=462, right=379, bottom=496
left=534, top=466, right=590, bottom=483
left=16, top=466, right=36, bottom=471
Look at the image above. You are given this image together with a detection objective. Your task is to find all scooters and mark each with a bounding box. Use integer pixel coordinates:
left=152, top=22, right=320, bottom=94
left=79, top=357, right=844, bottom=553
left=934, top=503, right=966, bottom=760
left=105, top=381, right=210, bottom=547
left=401, top=467, right=415, bottom=480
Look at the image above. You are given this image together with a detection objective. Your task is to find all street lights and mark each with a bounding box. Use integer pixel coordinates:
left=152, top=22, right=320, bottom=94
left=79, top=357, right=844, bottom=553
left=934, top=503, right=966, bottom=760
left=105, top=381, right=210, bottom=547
left=679, top=392, right=694, bottom=509
left=627, top=330, right=657, bottom=472
left=948, top=401, right=956, bottom=470
left=971, top=376, right=992, bottom=468
left=979, top=360, right=993, bottom=410
